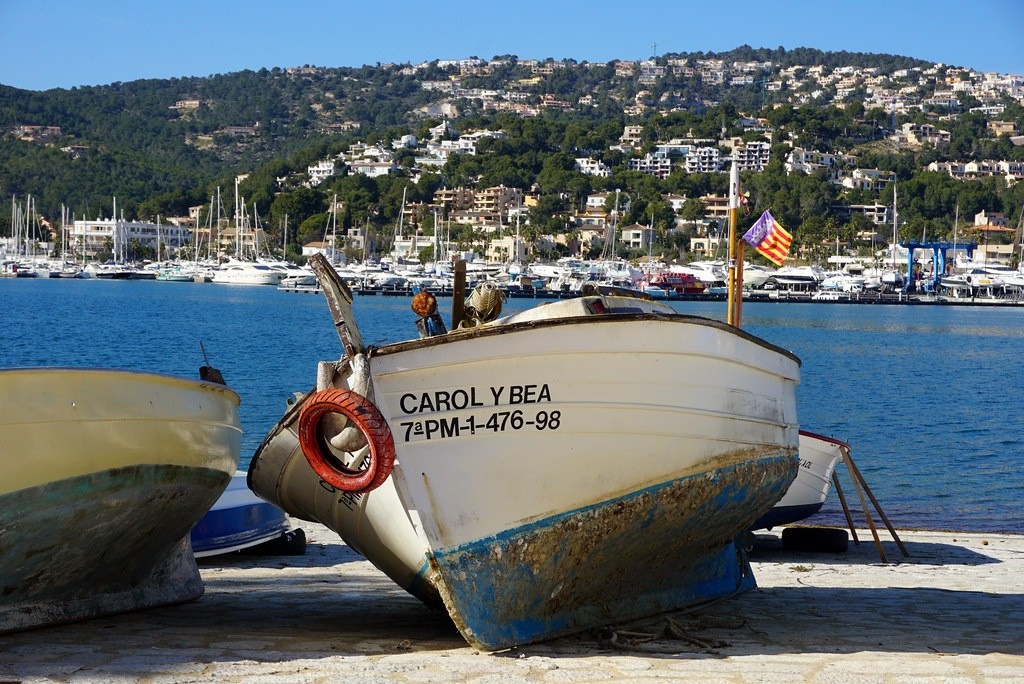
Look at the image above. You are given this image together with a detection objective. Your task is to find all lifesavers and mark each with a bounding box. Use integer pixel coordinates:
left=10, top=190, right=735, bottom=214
left=298, top=387, right=396, bottom=494
left=316, top=352, right=376, bottom=453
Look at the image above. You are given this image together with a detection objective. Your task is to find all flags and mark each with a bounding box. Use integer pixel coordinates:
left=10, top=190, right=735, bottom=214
left=744, top=210, right=793, bottom=267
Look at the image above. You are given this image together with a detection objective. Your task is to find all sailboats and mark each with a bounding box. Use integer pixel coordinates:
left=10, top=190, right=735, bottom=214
left=1, top=169, right=1023, bottom=307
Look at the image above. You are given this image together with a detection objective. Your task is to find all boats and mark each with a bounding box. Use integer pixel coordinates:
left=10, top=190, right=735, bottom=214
left=740, top=427, right=850, bottom=533
left=0, top=363, right=247, bottom=641
left=245, top=250, right=798, bottom=655
left=183, top=341, right=307, bottom=565
